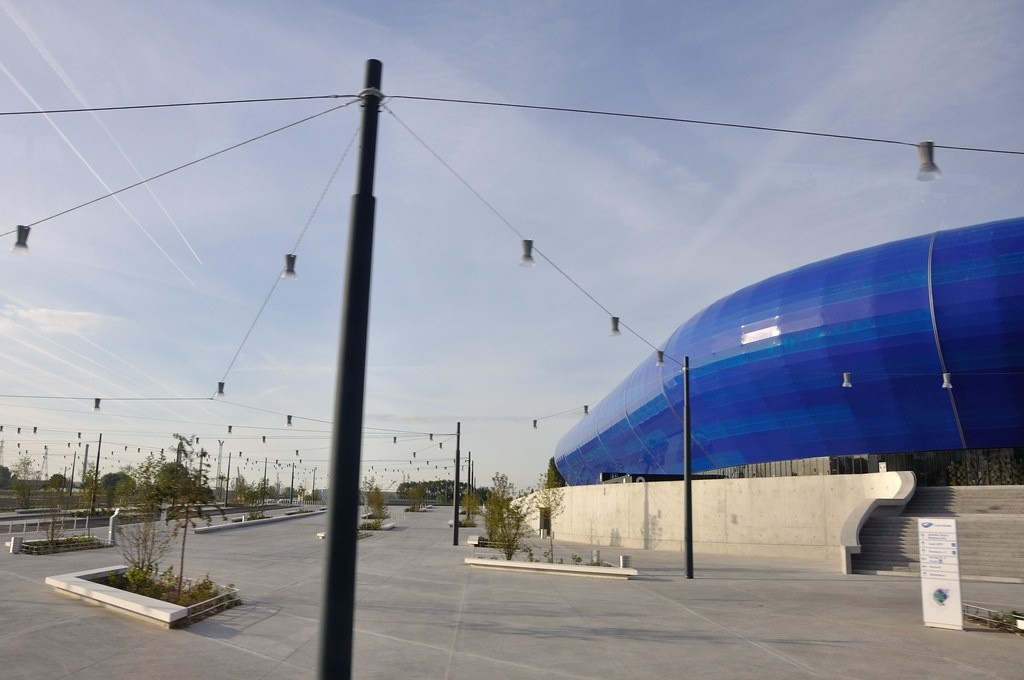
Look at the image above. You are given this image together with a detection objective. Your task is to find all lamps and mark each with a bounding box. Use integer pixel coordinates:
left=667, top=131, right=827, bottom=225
left=0, top=425, right=217, bottom=478
left=10, top=225, right=31, bottom=253
left=609, top=317, right=620, bottom=336
left=368, top=433, right=467, bottom=474
left=281, top=254, right=298, bottom=279
left=228, top=415, right=319, bottom=477
left=583, top=406, right=589, bottom=416
left=943, top=373, right=953, bottom=389
left=517, top=240, right=536, bottom=266
left=532, top=420, right=537, bottom=429
left=656, top=351, right=665, bottom=366
left=92, top=398, right=101, bottom=411
left=916, top=142, right=941, bottom=181
left=842, top=373, right=852, bottom=388
left=216, top=382, right=225, bottom=396
